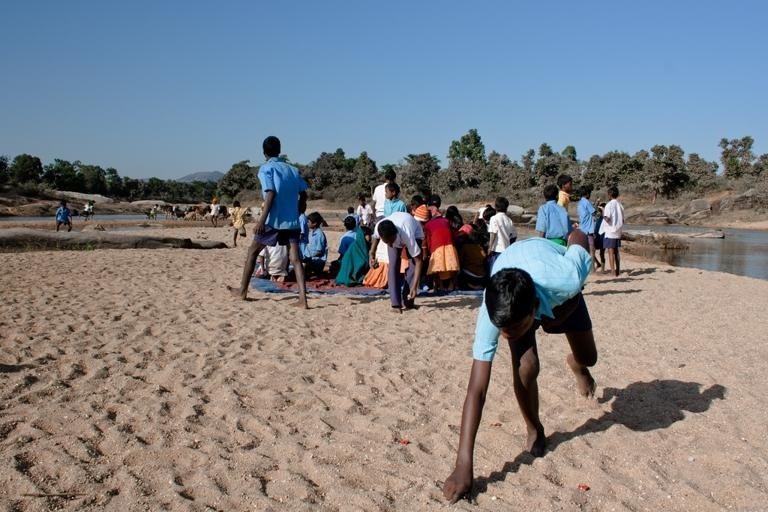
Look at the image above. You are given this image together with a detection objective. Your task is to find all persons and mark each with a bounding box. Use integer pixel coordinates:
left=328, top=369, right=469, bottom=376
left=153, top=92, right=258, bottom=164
left=442, top=229, right=597, bottom=504
left=56, top=200, right=95, bottom=232
